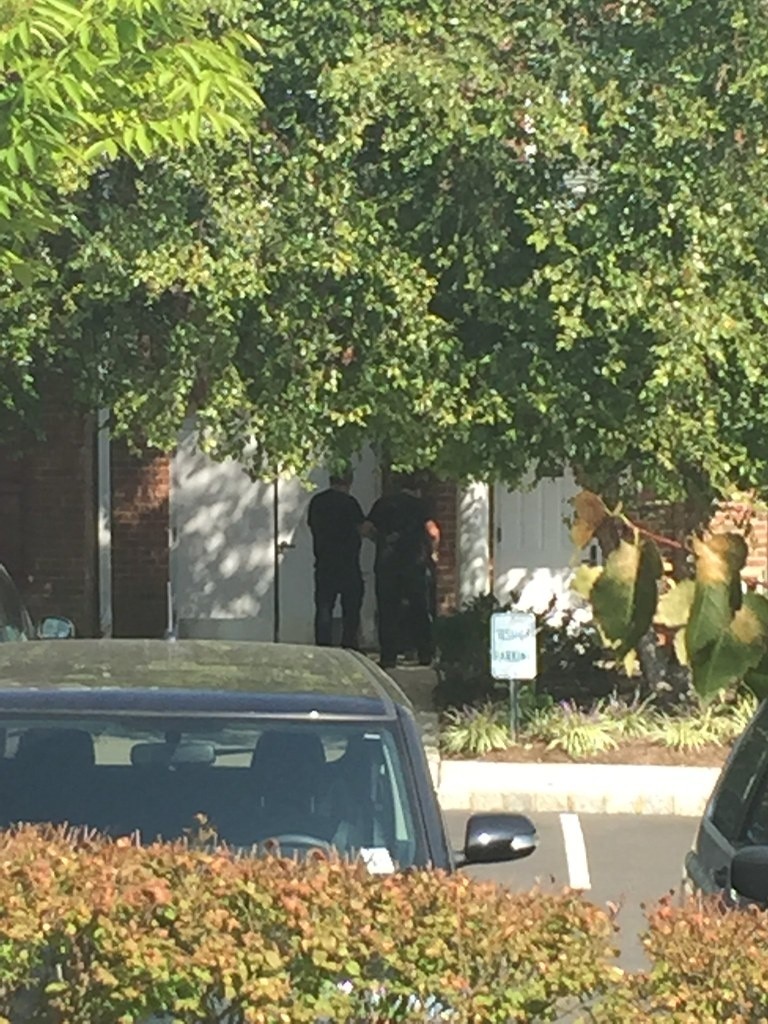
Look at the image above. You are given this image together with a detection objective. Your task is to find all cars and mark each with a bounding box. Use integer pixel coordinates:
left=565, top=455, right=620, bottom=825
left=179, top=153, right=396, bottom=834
left=683, top=695, right=768, bottom=914
left=0, top=562, right=76, bottom=644
left=0, top=636, right=543, bottom=882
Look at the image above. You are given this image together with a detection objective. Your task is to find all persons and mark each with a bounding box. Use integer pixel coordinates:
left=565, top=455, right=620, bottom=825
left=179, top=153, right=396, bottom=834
left=361, top=464, right=441, bottom=668
left=305, top=466, right=369, bottom=656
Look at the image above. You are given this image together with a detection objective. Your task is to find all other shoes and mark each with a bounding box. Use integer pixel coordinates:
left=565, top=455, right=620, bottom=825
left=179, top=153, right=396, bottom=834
left=419, top=655, right=431, bottom=665
left=376, top=659, right=395, bottom=669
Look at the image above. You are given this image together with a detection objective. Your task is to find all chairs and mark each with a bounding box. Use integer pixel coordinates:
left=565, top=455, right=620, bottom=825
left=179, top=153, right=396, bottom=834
left=250, top=729, right=329, bottom=781
left=17, top=726, right=98, bottom=772
